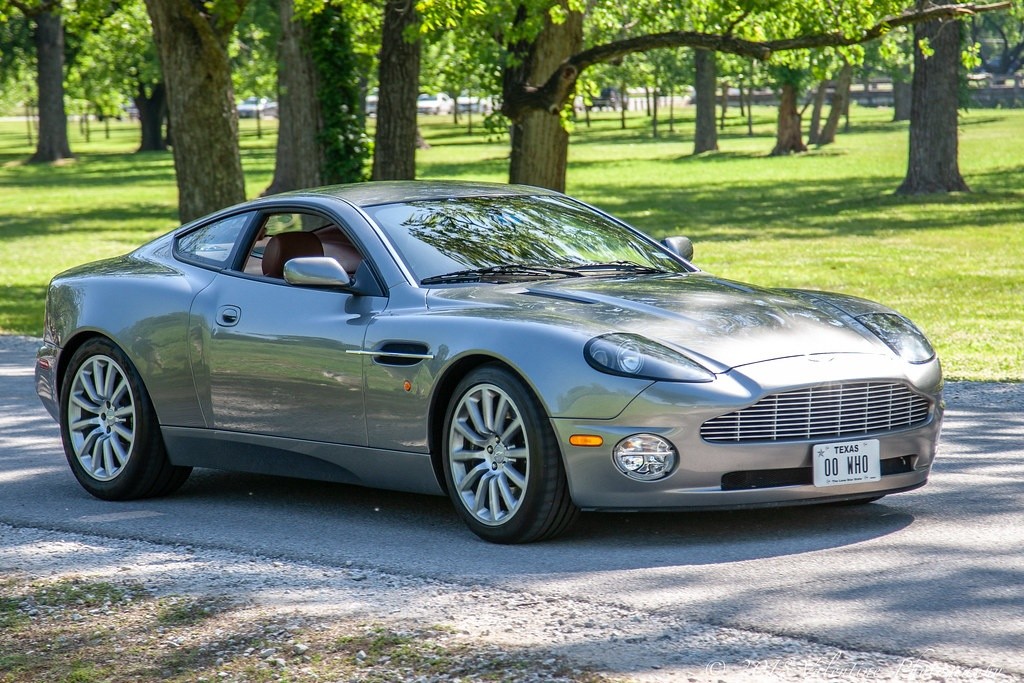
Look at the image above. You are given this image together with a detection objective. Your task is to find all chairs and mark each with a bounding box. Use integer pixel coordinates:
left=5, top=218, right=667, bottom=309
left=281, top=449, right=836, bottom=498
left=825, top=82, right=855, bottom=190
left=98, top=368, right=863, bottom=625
left=262, top=231, right=324, bottom=279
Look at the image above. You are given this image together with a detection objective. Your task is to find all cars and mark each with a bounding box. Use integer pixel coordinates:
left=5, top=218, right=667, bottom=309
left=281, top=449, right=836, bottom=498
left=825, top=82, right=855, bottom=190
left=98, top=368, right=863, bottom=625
left=587, top=87, right=616, bottom=110
left=365, top=84, right=503, bottom=115
left=32, top=179, right=945, bottom=545
left=236, top=95, right=278, bottom=119
